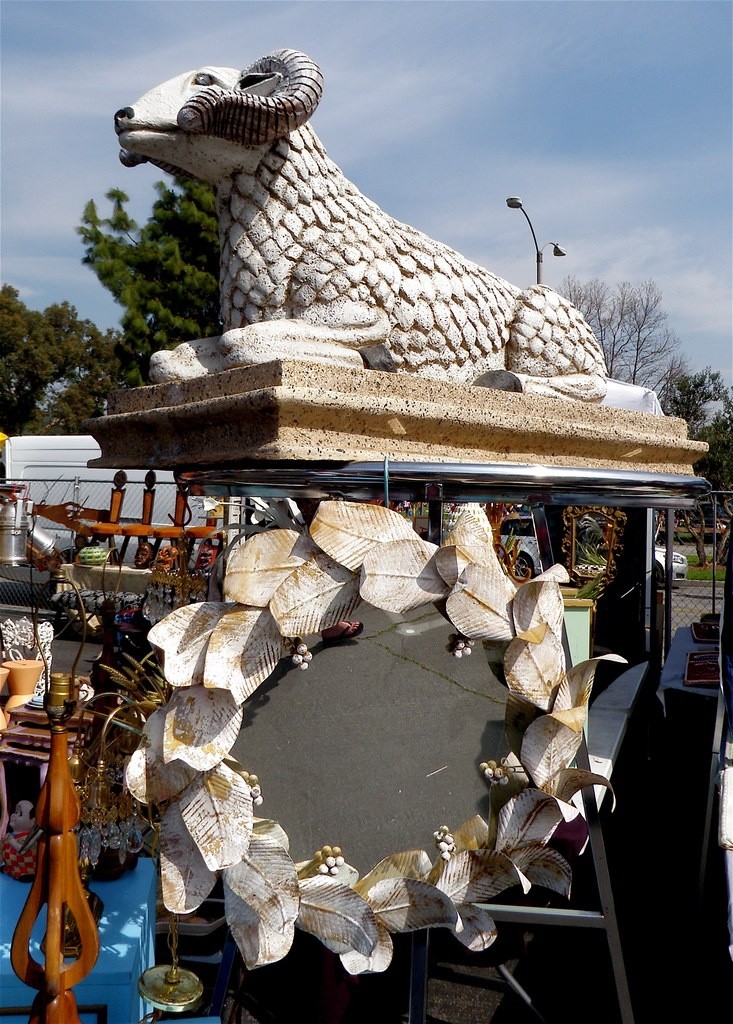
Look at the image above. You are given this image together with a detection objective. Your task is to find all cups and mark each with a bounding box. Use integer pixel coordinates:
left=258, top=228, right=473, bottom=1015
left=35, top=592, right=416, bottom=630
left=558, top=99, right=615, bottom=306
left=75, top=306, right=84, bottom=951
left=48, top=673, right=89, bottom=708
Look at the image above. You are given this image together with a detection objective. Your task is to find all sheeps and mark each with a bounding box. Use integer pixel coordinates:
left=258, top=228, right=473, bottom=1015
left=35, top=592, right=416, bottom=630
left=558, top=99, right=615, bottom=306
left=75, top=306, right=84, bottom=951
left=112, top=48, right=611, bottom=402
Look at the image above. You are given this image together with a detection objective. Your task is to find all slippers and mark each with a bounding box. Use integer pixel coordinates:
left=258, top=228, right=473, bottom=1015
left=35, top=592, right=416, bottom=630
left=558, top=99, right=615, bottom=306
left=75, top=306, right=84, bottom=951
left=322, top=620, right=363, bottom=646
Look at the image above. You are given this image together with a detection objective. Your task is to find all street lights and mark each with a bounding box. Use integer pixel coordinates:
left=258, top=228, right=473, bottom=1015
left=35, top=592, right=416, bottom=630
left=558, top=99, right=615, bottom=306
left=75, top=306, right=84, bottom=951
left=506, top=197, right=567, bottom=286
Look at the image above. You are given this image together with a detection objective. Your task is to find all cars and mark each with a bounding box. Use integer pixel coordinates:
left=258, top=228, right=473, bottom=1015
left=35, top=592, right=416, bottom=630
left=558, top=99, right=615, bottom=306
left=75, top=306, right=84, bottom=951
left=498, top=515, right=688, bottom=591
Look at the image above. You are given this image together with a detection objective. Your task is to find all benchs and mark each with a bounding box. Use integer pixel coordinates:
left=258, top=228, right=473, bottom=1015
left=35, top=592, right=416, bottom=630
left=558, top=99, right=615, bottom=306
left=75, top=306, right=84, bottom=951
left=569, top=660, right=650, bottom=855
left=45, top=588, right=143, bottom=638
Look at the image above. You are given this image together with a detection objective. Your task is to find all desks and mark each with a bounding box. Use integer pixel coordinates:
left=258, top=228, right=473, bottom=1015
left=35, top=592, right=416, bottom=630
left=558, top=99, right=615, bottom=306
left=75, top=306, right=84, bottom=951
left=656, top=628, right=720, bottom=719
left=55, top=562, right=177, bottom=634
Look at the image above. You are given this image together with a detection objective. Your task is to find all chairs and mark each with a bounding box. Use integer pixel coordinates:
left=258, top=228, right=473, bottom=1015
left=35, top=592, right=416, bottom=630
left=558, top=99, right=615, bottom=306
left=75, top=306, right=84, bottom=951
left=88, top=470, right=222, bottom=567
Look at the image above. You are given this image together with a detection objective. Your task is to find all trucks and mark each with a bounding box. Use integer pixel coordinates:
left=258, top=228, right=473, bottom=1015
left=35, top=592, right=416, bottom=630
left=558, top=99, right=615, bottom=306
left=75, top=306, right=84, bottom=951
left=4, top=433, right=308, bottom=572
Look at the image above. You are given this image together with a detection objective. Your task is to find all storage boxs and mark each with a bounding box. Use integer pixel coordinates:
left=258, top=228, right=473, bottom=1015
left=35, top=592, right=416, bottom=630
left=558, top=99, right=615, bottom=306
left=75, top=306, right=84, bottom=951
left=0, top=857, right=158, bottom=1024
